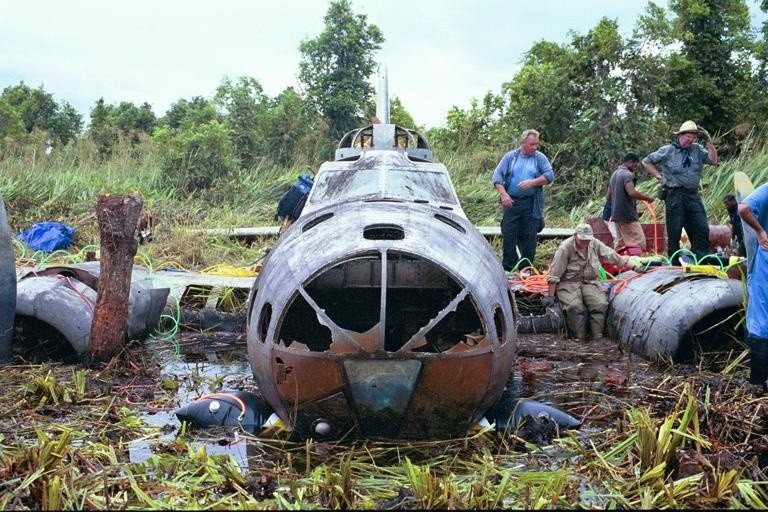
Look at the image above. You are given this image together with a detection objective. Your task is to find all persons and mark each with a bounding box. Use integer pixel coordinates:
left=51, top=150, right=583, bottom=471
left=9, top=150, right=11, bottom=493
left=548, top=224, right=645, bottom=343
left=642, top=120, right=719, bottom=265
left=491, top=127, right=555, bottom=272
left=605, top=152, right=655, bottom=252
left=723, top=194, right=746, bottom=258
left=736, top=180, right=768, bottom=384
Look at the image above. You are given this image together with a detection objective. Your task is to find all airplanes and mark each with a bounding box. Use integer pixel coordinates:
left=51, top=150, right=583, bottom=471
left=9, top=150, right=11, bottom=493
left=1, top=63, right=748, bottom=439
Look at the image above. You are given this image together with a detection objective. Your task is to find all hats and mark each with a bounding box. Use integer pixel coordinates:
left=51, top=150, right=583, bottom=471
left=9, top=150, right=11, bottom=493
left=673, top=120, right=703, bottom=136
left=574, top=224, right=593, bottom=241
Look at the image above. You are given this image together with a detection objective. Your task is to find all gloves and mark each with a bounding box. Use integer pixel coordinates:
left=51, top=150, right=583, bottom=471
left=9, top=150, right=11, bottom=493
left=632, top=264, right=646, bottom=274
left=657, top=173, right=667, bottom=187
left=541, top=296, right=555, bottom=307
left=697, top=125, right=711, bottom=143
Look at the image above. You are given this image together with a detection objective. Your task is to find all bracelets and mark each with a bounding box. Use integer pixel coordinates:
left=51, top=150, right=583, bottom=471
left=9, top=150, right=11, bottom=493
left=657, top=174, right=662, bottom=180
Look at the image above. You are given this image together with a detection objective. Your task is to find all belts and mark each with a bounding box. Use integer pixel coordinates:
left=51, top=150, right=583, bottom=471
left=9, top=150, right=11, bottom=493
left=510, top=195, right=535, bottom=200
